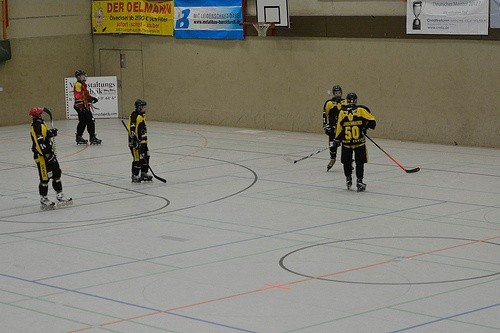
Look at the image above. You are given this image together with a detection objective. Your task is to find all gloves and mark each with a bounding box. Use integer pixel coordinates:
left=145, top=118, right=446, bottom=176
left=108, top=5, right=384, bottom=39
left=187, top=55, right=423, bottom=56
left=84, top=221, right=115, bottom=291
left=49, top=128, right=58, bottom=137
left=82, top=108, right=88, bottom=114
left=333, top=141, right=340, bottom=147
left=92, top=98, right=98, bottom=103
left=360, top=126, right=366, bottom=135
left=132, top=141, right=139, bottom=150
left=325, top=128, right=332, bottom=135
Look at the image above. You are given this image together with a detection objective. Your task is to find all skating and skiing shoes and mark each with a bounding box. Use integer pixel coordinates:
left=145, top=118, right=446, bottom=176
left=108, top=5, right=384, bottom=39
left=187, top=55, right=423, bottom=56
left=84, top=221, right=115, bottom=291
left=357, top=179, right=366, bottom=192
left=75, top=133, right=88, bottom=145
left=346, top=177, right=352, bottom=190
left=89, top=137, right=102, bottom=145
left=141, top=175, right=153, bottom=182
left=56, top=192, right=73, bottom=207
left=40, top=198, right=56, bottom=211
left=132, top=175, right=143, bottom=184
left=326, top=159, right=335, bottom=172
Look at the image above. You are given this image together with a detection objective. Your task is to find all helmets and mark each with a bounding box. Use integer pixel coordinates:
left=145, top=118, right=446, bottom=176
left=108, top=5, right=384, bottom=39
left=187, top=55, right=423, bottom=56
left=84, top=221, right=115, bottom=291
left=332, top=85, right=342, bottom=95
left=29, top=106, right=44, bottom=119
left=346, top=92, right=357, bottom=104
left=75, top=70, right=85, bottom=77
left=135, top=99, right=147, bottom=107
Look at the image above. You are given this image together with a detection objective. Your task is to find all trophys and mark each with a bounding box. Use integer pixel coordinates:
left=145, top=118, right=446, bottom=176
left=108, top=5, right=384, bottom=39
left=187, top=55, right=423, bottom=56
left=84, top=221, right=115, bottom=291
left=409, top=0, right=426, bottom=30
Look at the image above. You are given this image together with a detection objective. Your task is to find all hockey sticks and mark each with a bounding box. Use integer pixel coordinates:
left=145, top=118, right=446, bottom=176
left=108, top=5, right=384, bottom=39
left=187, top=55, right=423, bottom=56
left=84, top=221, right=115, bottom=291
left=43, top=107, right=54, bottom=151
left=121, top=119, right=166, bottom=183
left=294, top=146, right=330, bottom=164
left=366, top=133, right=420, bottom=173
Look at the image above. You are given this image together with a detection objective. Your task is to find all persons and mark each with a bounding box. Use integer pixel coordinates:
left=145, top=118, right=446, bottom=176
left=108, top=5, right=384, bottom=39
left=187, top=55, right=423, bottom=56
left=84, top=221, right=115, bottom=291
left=127, top=99, right=153, bottom=183
left=322, top=84, right=354, bottom=172
left=73, top=70, right=103, bottom=146
left=334, top=92, right=376, bottom=192
left=29, top=106, right=74, bottom=210
